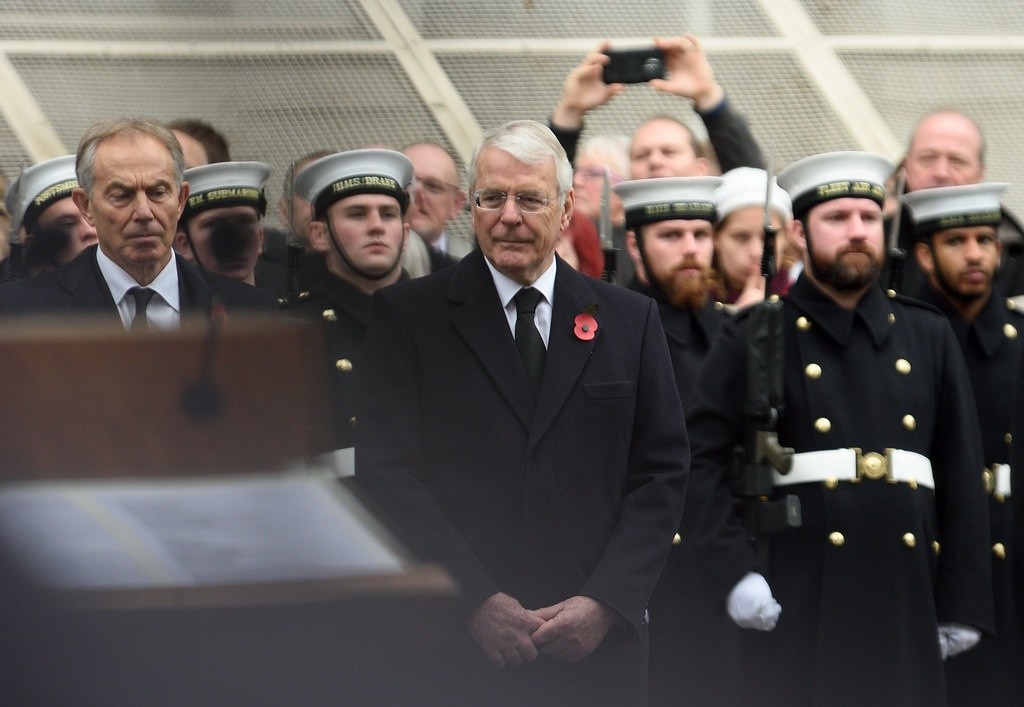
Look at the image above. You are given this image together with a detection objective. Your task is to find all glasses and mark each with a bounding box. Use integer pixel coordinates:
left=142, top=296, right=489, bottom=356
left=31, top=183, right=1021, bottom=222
left=470, top=188, right=562, bottom=214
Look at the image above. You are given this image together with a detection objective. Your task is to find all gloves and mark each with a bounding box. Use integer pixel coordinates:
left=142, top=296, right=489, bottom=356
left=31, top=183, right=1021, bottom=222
left=938, top=620, right=982, bottom=659
left=725, top=572, right=782, bottom=630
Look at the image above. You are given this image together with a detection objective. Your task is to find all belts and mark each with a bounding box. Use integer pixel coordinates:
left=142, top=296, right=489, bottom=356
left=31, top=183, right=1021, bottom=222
left=984, top=462, right=1011, bottom=494
left=769, top=448, right=936, bottom=490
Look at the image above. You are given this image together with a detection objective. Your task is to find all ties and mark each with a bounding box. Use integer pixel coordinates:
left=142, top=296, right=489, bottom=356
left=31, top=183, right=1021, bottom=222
left=127, top=286, right=154, bottom=338
left=513, top=287, right=548, bottom=406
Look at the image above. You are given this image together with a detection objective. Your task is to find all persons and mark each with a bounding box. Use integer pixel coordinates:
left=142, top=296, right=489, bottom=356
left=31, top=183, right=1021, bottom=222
left=0, top=32, right=1023, bottom=707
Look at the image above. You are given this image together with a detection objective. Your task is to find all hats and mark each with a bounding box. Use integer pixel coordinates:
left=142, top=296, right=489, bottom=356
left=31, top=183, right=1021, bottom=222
left=294, top=148, right=414, bottom=221
left=777, top=150, right=896, bottom=218
left=610, top=176, right=724, bottom=228
left=900, top=182, right=1006, bottom=233
left=713, top=166, right=793, bottom=226
left=5, top=153, right=81, bottom=233
left=178, top=161, right=270, bottom=223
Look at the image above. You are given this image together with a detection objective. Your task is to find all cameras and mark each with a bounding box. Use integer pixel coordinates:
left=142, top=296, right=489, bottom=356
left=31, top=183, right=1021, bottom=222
left=600, top=49, right=667, bottom=85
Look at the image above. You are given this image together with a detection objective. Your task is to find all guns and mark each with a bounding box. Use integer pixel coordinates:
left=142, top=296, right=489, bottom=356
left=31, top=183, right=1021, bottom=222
left=725, top=153, right=793, bottom=634
left=877, top=167, right=912, bottom=291
left=276, top=160, right=306, bottom=306
left=595, top=165, right=622, bottom=291
